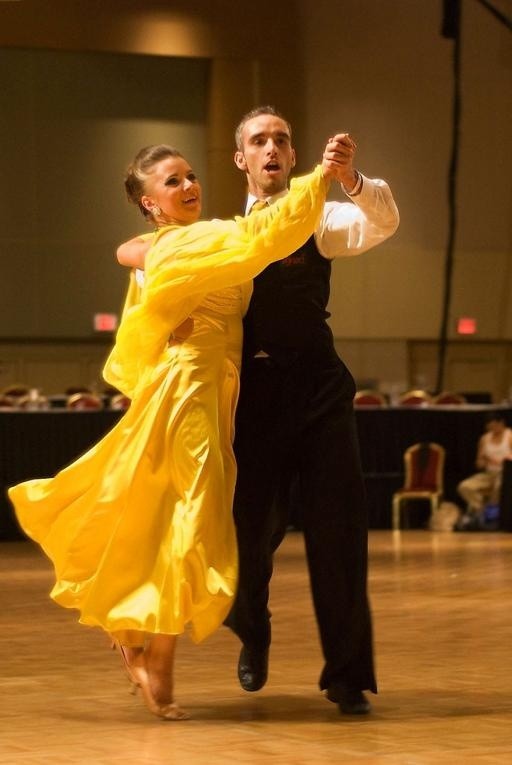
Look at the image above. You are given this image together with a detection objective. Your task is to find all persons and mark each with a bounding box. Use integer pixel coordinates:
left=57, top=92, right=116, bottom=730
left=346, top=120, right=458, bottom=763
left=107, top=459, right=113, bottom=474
left=6, top=127, right=361, bottom=725
left=456, top=411, right=511, bottom=531
left=157, top=101, right=404, bottom=717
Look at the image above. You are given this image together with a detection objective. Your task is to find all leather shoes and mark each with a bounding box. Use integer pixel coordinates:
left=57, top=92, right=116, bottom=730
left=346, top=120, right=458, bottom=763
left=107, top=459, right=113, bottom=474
left=324, top=688, right=371, bottom=718
left=237, top=640, right=270, bottom=692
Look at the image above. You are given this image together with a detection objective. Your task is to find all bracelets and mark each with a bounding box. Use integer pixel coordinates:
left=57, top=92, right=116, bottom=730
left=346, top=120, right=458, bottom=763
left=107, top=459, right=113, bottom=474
left=344, top=166, right=362, bottom=195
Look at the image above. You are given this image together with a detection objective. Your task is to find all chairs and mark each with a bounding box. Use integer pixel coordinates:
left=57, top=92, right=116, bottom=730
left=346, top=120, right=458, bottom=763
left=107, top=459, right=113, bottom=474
left=1, top=385, right=466, bottom=408
left=392, top=441, right=446, bottom=532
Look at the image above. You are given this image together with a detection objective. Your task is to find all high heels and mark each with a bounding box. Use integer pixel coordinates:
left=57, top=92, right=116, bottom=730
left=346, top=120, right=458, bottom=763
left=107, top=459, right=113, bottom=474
left=111, top=634, right=191, bottom=722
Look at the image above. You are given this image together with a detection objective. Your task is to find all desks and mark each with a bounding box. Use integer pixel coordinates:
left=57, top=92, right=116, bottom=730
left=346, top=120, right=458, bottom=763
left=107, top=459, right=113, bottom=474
left=0, top=408, right=512, bottom=543
